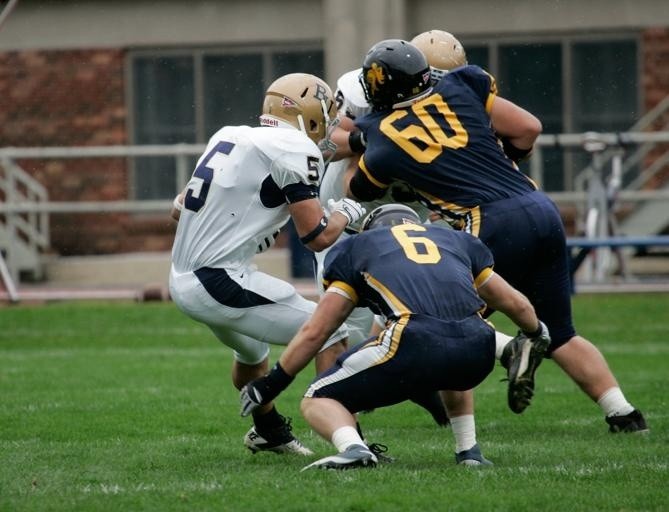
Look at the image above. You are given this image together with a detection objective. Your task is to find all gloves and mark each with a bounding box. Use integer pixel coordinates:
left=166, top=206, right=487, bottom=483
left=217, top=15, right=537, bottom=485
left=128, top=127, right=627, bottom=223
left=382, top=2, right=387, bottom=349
left=238, top=361, right=294, bottom=417
left=522, top=320, right=551, bottom=357
left=330, top=197, right=367, bottom=226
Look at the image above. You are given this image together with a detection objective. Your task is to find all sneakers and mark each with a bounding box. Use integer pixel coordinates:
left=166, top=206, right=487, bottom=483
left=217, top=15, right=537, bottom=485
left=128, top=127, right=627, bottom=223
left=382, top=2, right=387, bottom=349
left=501, top=331, right=547, bottom=414
left=454, top=444, right=495, bottom=467
left=605, top=409, right=652, bottom=436
left=299, top=445, right=378, bottom=471
left=244, top=427, right=313, bottom=457
left=364, top=443, right=399, bottom=464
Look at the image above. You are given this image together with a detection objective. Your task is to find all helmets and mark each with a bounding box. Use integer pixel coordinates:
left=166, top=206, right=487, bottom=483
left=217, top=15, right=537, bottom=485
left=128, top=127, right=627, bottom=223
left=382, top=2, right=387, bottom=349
left=357, top=39, right=434, bottom=110
left=360, top=204, right=422, bottom=231
left=410, top=30, right=468, bottom=86
left=263, top=72, right=340, bottom=153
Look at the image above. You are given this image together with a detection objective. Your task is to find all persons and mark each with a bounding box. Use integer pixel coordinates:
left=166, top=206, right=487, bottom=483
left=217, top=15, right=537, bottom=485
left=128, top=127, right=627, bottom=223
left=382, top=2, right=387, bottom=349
left=313, top=29, right=467, bottom=352
left=343, top=40, right=650, bottom=435
left=240, top=202, right=551, bottom=474
left=168, top=73, right=399, bottom=468
